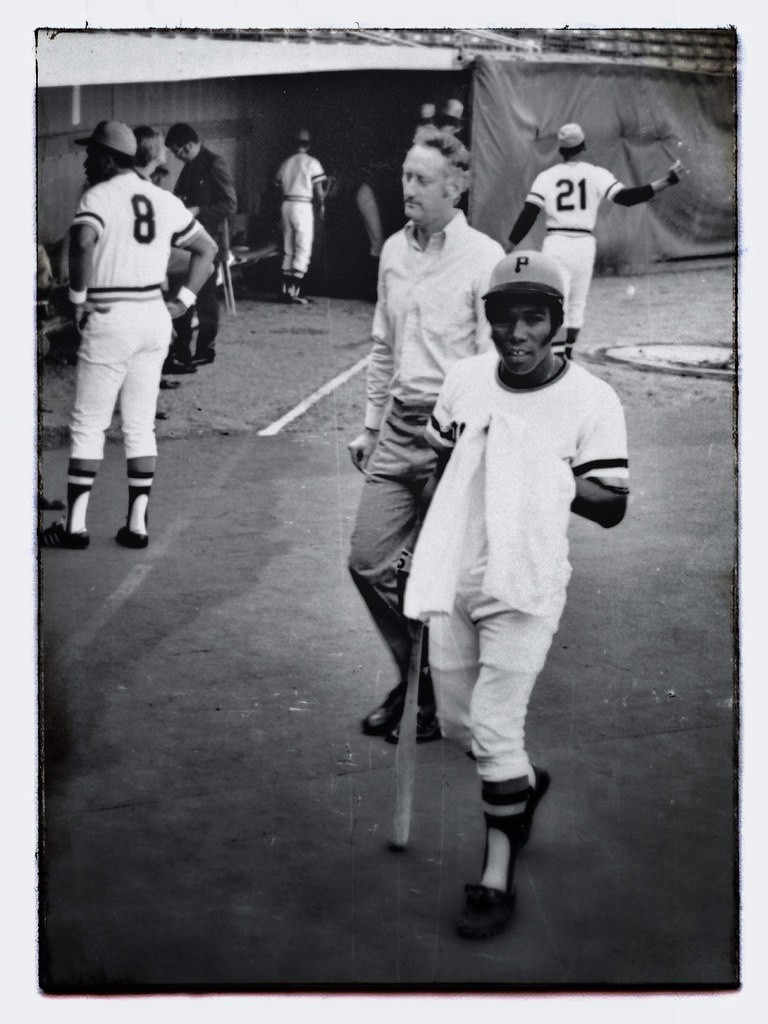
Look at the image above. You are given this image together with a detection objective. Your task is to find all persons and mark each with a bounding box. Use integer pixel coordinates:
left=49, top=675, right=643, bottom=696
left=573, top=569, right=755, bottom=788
left=346, top=124, right=510, bottom=748
left=402, top=250, right=639, bottom=937
left=36, top=118, right=693, bottom=552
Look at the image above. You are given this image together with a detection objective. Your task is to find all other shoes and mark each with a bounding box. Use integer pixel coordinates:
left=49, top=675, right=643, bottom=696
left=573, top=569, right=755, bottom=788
left=41, top=521, right=89, bottom=549
left=191, top=351, right=213, bottom=367
left=512, top=764, right=550, bottom=849
left=163, top=357, right=196, bottom=375
left=117, top=527, right=149, bottom=548
left=386, top=701, right=443, bottom=745
left=458, top=882, right=516, bottom=939
left=279, top=292, right=313, bottom=304
left=363, top=677, right=429, bottom=735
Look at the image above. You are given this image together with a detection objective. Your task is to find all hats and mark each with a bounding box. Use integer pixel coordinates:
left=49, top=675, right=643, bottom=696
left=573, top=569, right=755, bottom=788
left=559, top=122, right=584, bottom=149
left=482, top=250, right=566, bottom=308
left=74, top=120, right=138, bottom=156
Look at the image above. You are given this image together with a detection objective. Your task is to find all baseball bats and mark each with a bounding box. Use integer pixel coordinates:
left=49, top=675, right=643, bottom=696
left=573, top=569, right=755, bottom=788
left=221, top=257, right=240, bottom=317
left=385, top=622, right=426, bottom=848
